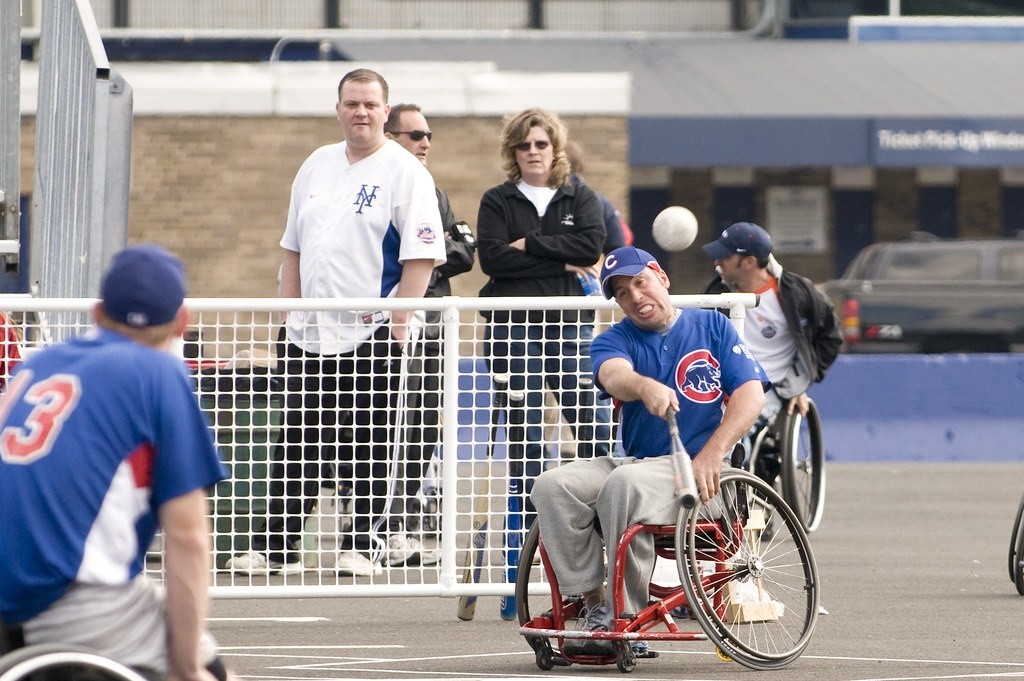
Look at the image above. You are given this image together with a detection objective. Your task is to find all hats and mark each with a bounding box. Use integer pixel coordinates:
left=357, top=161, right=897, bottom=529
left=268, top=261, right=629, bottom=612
left=700, top=221, right=772, bottom=269
left=600, top=245, right=657, bottom=300
left=99, top=241, right=187, bottom=329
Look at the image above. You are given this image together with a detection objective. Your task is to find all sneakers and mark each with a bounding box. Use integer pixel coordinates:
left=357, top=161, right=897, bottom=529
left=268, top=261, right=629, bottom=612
left=407, top=536, right=442, bottom=566
left=381, top=523, right=422, bottom=566
left=562, top=592, right=617, bottom=656
left=334, top=548, right=383, bottom=576
left=225, top=548, right=303, bottom=576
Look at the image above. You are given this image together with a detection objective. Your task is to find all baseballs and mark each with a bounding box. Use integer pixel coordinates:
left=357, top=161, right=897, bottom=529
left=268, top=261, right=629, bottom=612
left=651, top=205, right=700, bottom=252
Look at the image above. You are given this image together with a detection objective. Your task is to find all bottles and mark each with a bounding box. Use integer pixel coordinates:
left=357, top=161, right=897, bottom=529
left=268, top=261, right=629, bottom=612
left=864, top=324, right=902, bottom=339
left=842, top=300, right=859, bottom=343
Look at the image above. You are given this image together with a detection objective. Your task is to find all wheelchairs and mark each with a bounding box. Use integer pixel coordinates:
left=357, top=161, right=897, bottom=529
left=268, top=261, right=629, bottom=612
left=0, top=615, right=227, bottom=681
left=516, top=391, right=820, bottom=673
left=1008, top=493, right=1024, bottom=595
left=737, top=395, right=826, bottom=540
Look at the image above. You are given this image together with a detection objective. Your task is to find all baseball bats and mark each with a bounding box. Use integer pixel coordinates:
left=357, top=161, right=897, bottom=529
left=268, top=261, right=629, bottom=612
left=667, top=404, right=699, bottom=509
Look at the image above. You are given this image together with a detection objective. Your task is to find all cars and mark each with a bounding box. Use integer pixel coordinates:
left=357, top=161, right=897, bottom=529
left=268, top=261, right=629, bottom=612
left=820, top=235, right=1024, bottom=354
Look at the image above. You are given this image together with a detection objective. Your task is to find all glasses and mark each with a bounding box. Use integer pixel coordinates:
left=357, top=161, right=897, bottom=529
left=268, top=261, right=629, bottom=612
left=515, top=140, right=550, bottom=151
left=392, top=130, right=432, bottom=142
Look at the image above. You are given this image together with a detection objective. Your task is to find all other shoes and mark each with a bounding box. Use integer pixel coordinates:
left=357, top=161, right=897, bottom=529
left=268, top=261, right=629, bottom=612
left=756, top=520, right=773, bottom=541
left=518, top=549, right=542, bottom=567
left=620, top=612, right=650, bottom=655
left=671, top=603, right=696, bottom=620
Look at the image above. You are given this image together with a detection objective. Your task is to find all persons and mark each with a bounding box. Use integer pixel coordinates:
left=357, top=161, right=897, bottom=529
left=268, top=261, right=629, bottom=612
left=225, top=68, right=476, bottom=575
left=477, top=107, right=625, bottom=565
left=701, top=222, right=844, bottom=501
left=0, top=248, right=226, bottom=681
left=530, top=245, right=770, bottom=653
